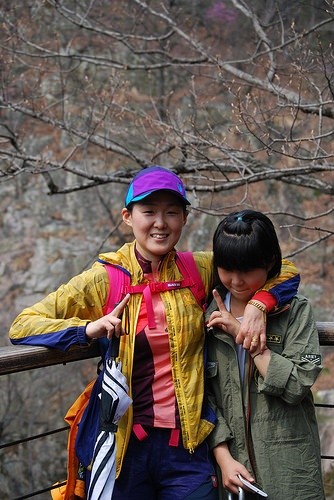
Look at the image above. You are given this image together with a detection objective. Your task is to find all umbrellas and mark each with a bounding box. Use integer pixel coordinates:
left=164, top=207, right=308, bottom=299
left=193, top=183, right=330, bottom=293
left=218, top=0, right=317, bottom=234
left=86, top=302, right=133, bottom=500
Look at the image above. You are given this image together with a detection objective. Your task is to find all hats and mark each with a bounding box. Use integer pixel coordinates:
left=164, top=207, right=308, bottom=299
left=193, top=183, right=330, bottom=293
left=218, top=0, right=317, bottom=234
left=125, top=167, right=193, bottom=210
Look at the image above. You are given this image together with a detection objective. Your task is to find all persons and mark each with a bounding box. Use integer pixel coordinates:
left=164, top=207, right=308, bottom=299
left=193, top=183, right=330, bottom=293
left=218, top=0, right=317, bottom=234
left=203, top=210, right=326, bottom=500
left=9, top=166, right=302, bottom=500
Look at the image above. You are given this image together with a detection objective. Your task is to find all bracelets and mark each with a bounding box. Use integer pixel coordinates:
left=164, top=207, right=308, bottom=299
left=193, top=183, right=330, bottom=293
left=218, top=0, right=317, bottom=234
left=247, top=300, right=268, bottom=314
left=253, top=347, right=270, bottom=359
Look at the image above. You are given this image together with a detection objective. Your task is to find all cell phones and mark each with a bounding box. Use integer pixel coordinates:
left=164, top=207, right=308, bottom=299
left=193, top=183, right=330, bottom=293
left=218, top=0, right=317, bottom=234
left=238, top=474, right=268, bottom=497
